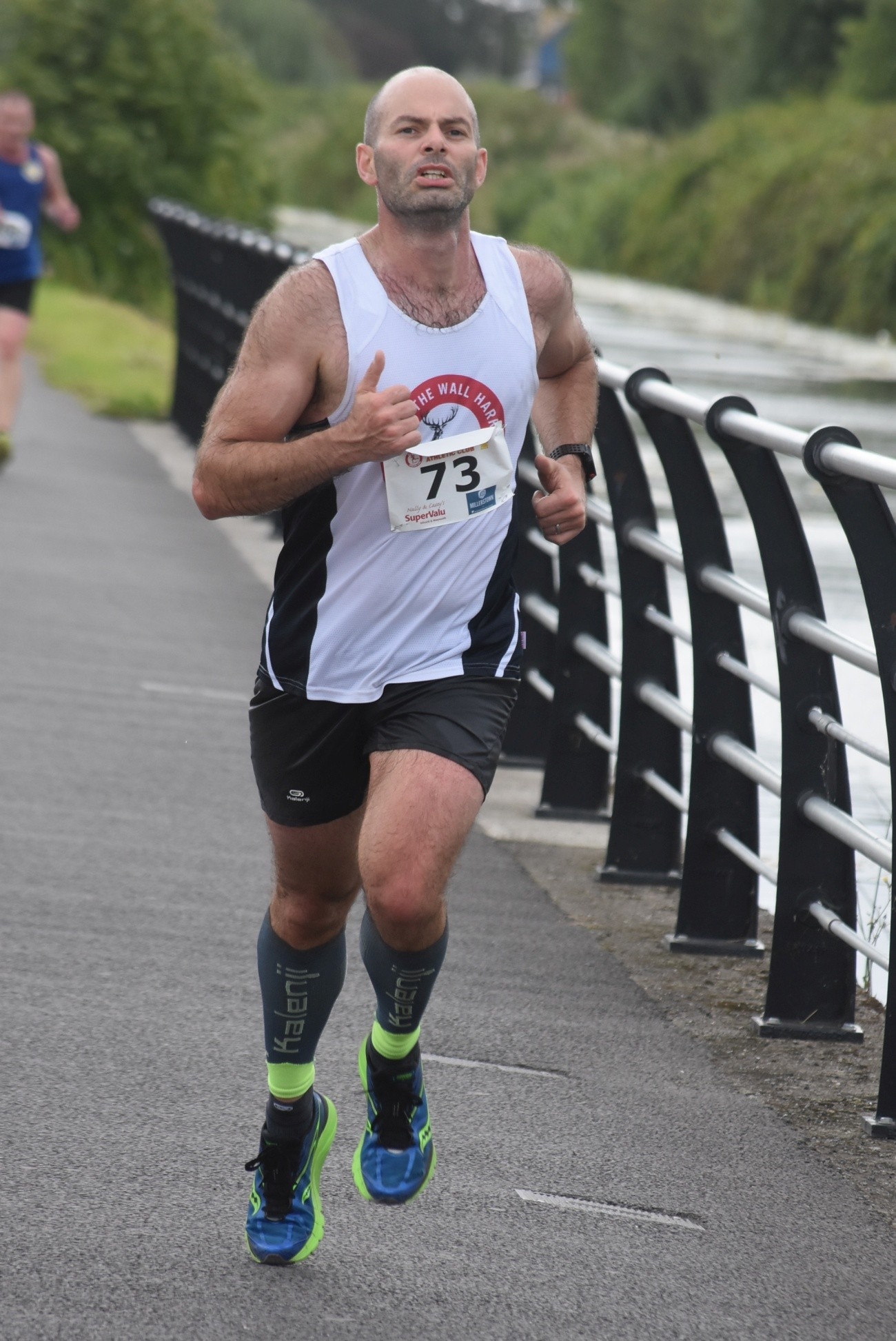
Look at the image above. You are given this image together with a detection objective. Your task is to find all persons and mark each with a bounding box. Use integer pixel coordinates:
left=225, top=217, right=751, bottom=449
left=190, top=65, right=600, bottom=1265
left=0, top=89, right=79, bottom=465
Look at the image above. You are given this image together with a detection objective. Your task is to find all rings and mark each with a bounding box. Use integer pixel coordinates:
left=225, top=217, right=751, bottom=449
left=555, top=524, right=560, bottom=533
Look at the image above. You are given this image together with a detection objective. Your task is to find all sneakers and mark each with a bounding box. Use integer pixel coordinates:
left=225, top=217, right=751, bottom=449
left=245, top=1091, right=338, bottom=1267
left=352, top=1031, right=437, bottom=1205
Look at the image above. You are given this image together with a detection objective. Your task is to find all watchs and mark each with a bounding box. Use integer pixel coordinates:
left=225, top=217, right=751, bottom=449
left=549, top=443, right=597, bottom=482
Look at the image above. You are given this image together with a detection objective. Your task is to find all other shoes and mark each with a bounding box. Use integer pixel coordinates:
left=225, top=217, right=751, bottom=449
left=0, top=430, right=14, bottom=468
